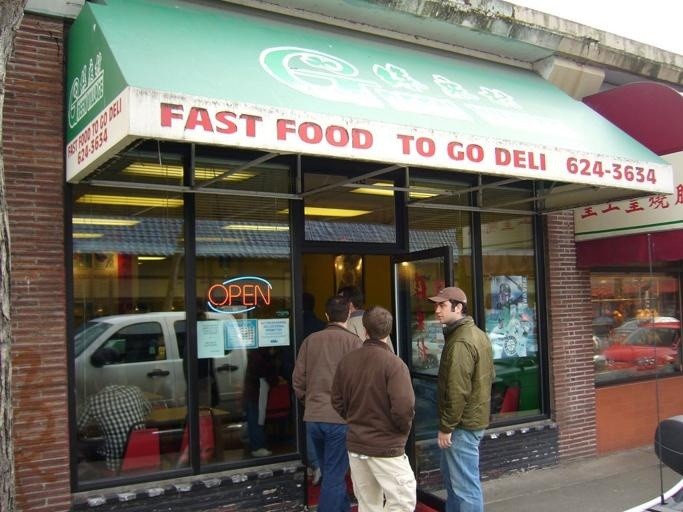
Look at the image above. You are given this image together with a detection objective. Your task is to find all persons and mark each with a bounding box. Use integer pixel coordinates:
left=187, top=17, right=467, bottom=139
left=183, top=310, right=225, bottom=464
left=337, top=286, right=394, bottom=352
left=292, top=297, right=367, bottom=511
left=75, top=371, right=153, bottom=479
left=332, top=307, right=419, bottom=511
left=302, top=292, right=327, bottom=339
left=427, top=287, right=496, bottom=512
left=244, top=304, right=284, bottom=457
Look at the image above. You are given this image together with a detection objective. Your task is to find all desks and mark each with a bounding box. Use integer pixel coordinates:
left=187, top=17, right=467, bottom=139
left=142, top=404, right=232, bottom=428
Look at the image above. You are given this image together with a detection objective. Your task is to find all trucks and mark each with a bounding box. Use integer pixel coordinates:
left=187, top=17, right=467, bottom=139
left=73, top=311, right=288, bottom=445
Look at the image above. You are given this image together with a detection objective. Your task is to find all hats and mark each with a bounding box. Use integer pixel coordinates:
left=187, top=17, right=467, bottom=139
left=426, top=286, right=469, bottom=307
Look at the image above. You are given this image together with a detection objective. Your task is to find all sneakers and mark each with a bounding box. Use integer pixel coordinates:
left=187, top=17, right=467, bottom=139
left=250, top=447, right=275, bottom=458
left=307, top=467, right=323, bottom=488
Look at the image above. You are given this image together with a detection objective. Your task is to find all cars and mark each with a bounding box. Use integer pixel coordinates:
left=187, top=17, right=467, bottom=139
left=602, top=319, right=680, bottom=370
left=592, top=314, right=679, bottom=353
left=407, top=317, right=539, bottom=368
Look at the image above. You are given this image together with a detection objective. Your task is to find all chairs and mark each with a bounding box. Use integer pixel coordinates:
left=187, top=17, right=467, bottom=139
left=101, top=417, right=161, bottom=480
left=497, top=378, right=521, bottom=417
left=167, top=405, right=219, bottom=469
